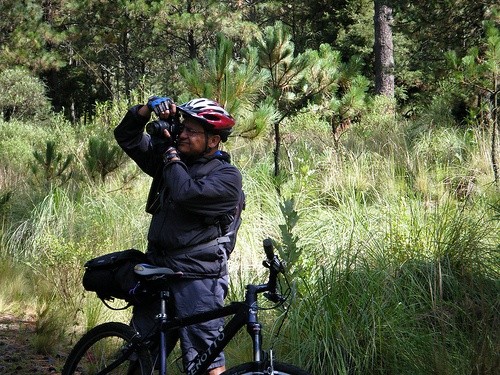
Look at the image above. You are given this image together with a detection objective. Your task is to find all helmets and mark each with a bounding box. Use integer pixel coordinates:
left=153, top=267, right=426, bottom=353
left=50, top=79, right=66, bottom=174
left=176, top=97, right=235, bottom=143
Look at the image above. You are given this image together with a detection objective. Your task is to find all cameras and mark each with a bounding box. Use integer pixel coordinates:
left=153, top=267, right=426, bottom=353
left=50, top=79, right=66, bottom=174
left=146, top=111, right=180, bottom=144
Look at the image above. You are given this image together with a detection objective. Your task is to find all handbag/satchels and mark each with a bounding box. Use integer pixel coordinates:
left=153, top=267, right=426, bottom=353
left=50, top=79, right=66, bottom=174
left=83, top=248, right=150, bottom=309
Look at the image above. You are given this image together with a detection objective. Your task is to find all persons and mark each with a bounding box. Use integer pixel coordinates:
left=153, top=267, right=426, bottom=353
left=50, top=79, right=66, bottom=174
left=113, top=95, right=245, bottom=375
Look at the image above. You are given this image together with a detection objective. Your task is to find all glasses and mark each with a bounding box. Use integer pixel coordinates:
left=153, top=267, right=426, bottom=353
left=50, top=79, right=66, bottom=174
left=180, top=125, right=206, bottom=139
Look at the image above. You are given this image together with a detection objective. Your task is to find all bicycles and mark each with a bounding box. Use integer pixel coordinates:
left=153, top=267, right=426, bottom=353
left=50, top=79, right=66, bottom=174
left=61, top=238, right=313, bottom=375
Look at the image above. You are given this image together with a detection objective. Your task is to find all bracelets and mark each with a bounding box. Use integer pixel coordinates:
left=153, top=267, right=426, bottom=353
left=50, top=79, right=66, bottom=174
left=163, top=153, right=179, bottom=163
